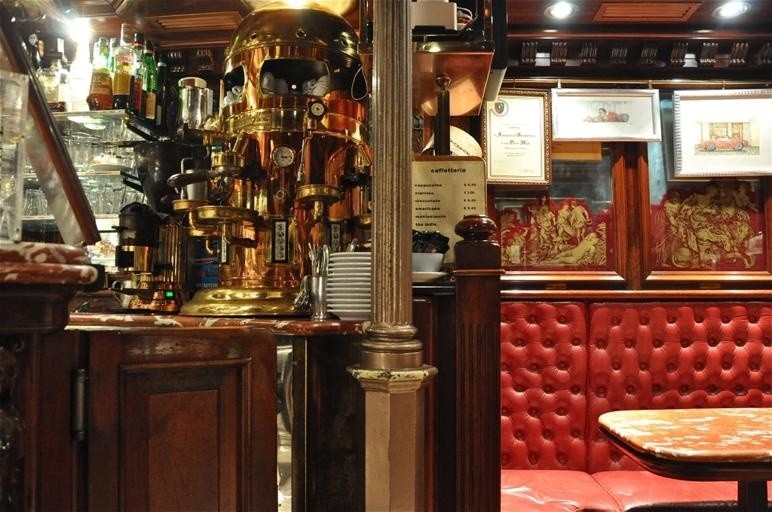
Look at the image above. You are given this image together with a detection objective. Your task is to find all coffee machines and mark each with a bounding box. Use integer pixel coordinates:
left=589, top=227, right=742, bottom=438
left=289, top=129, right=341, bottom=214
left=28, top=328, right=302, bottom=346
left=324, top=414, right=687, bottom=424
left=109, top=202, right=183, bottom=314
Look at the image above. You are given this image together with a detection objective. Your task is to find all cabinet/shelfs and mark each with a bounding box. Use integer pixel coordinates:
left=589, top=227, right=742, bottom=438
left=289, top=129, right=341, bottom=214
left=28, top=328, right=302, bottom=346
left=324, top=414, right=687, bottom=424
left=86, top=332, right=308, bottom=512
left=1, top=279, right=83, bottom=512
left=638, top=95, right=767, bottom=286
left=28, top=104, right=162, bottom=243
left=489, top=139, right=629, bottom=284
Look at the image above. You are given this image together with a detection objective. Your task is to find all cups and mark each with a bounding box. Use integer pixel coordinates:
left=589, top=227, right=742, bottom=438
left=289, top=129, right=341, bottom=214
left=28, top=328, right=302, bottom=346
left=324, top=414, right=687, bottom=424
left=186, top=169, right=208, bottom=200
left=23, top=189, right=41, bottom=215
left=78, top=174, right=149, bottom=211
left=61, top=121, right=138, bottom=170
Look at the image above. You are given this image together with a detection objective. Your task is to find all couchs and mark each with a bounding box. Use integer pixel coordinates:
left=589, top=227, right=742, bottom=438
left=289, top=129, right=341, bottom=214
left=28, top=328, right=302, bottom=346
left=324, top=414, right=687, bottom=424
left=586, top=303, right=770, bottom=510
left=499, top=298, right=625, bottom=512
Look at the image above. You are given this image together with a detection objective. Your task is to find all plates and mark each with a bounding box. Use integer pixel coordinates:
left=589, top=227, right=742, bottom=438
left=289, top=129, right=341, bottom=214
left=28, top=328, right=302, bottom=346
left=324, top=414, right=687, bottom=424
left=172, top=199, right=210, bottom=211
left=295, top=184, right=342, bottom=201
left=324, top=252, right=372, bottom=320
left=411, top=272, right=448, bottom=281
left=196, top=206, right=251, bottom=222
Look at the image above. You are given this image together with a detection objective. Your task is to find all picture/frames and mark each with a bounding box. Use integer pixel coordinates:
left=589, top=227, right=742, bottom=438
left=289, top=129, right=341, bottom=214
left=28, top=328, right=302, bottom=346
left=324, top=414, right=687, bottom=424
left=549, top=86, right=662, bottom=145
left=481, top=86, right=552, bottom=190
left=673, top=85, right=772, bottom=179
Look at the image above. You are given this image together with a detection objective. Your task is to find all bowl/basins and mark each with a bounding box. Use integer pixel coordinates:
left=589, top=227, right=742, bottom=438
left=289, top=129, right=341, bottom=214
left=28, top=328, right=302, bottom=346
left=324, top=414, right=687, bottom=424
left=412, top=253, right=443, bottom=272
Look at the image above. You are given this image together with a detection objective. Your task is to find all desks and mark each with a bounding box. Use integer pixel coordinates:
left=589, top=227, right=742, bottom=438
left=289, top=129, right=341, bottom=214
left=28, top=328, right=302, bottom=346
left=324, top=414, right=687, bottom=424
left=595, top=404, right=772, bottom=511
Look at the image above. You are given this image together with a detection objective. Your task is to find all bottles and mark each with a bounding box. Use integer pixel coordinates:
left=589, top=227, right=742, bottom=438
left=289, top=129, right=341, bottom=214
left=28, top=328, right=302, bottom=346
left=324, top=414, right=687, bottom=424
left=155, top=61, right=169, bottom=132
left=113, top=23, right=137, bottom=110
left=88, top=36, right=114, bottom=110
left=132, top=31, right=149, bottom=118
left=144, top=39, right=159, bottom=121
left=109, top=37, right=118, bottom=75
